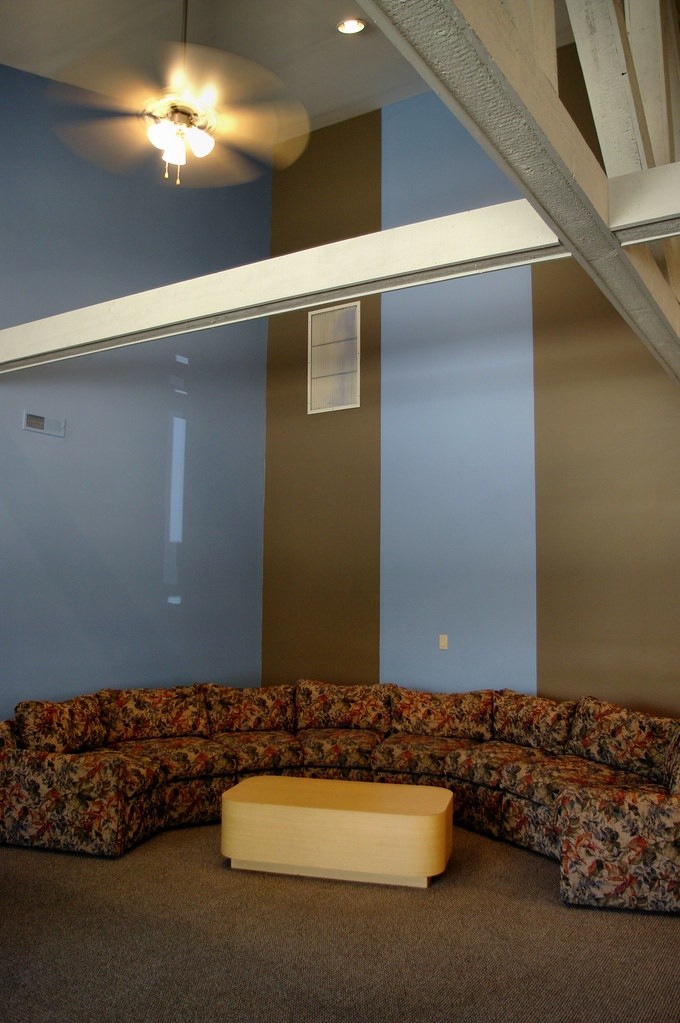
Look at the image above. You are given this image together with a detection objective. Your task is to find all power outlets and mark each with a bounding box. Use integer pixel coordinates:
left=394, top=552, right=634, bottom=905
left=439, top=635, right=448, bottom=650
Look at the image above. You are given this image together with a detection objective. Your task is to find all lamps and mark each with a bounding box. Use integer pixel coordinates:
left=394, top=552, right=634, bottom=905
left=147, top=120, right=214, bottom=165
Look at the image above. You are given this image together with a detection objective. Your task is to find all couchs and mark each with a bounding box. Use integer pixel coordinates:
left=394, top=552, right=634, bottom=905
left=0, top=722, right=680, bottom=911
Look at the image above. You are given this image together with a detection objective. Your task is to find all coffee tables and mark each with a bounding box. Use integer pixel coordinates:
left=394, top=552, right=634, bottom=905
left=221, top=775, right=454, bottom=889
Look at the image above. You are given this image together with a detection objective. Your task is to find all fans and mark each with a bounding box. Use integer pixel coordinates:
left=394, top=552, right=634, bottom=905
left=50, top=0, right=311, bottom=187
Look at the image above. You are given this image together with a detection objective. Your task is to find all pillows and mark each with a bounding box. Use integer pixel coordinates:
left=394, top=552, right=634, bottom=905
left=566, top=694, right=672, bottom=782
left=494, top=689, right=575, bottom=755
left=13, top=694, right=106, bottom=752
left=390, top=685, right=493, bottom=742
left=96, top=684, right=212, bottom=743
left=297, top=678, right=400, bottom=732
left=666, top=727, right=680, bottom=795
left=198, top=684, right=296, bottom=731
